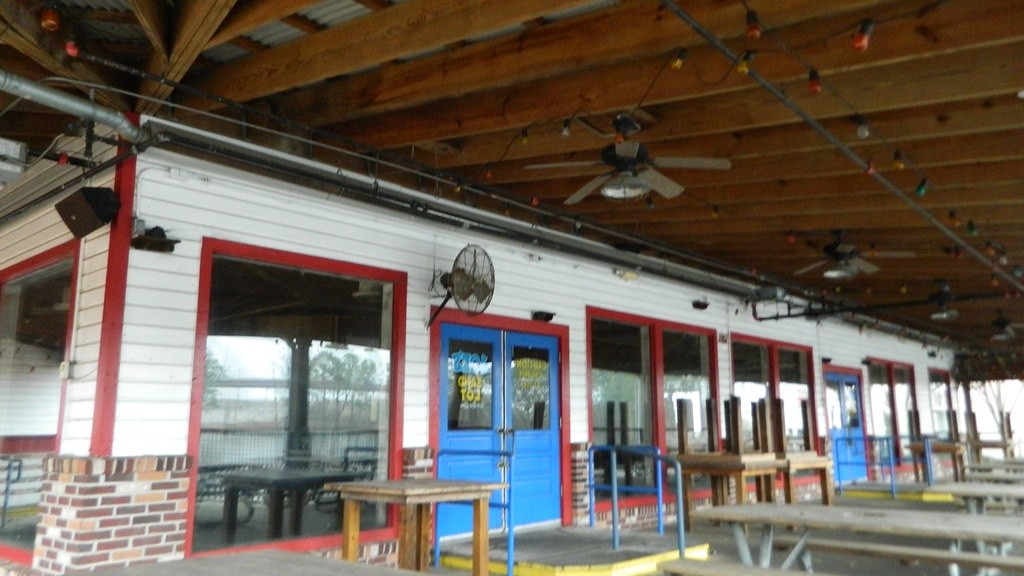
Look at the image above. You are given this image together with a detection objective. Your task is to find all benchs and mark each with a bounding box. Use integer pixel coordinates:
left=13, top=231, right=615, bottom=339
left=659, top=503, right=1024, bottom=575
left=931, top=461, right=1024, bottom=514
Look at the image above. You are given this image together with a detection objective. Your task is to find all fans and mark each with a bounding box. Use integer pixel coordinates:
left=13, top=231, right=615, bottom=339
left=804, top=242, right=881, bottom=285
left=525, top=114, right=733, bottom=205
left=442, top=244, right=495, bottom=316
left=924, top=282, right=971, bottom=325
left=986, top=312, right=1024, bottom=342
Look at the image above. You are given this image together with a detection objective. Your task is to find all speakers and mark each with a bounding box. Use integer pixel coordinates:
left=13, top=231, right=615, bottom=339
left=55, top=187, right=122, bottom=239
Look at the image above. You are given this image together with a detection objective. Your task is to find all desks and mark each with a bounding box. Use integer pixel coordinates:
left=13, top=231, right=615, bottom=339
left=224, top=469, right=356, bottom=543
left=751, top=397, right=829, bottom=464
left=677, top=395, right=787, bottom=465
left=907, top=407, right=1016, bottom=480
left=674, top=463, right=775, bottom=535
left=323, top=479, right=509, bottom=576
left=755, top=463, right=834, bottom=508
